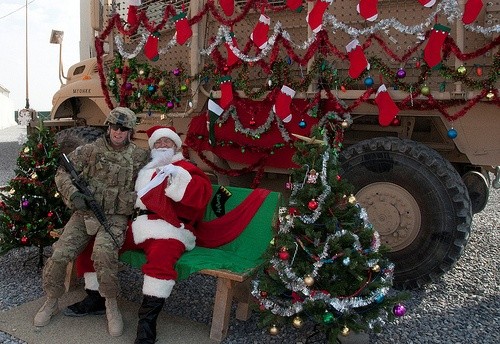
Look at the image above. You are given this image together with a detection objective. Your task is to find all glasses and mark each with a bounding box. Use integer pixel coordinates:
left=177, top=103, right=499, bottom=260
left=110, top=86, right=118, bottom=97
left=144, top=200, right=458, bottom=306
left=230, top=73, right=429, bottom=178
left=110, top=125, right=130, bottom=132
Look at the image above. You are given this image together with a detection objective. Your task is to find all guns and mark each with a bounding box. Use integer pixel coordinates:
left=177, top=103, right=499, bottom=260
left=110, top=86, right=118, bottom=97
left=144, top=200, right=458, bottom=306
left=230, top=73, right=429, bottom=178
left=61, top=152, right=122, bottom=249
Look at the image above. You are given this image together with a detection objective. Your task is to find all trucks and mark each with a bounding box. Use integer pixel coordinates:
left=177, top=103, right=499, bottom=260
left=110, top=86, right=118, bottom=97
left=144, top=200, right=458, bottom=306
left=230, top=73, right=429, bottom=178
left=37, top=0, right=500, bottom=292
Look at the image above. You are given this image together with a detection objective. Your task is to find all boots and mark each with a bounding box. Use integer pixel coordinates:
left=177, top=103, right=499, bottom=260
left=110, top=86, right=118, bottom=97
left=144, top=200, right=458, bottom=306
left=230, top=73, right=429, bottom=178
left=104, top=297, right=124, bottom=337
left=33, top=296, right=60, bottom=327
left=133, top=295, right=166, bottom=344
left=64, top=289, right=107, bottom=316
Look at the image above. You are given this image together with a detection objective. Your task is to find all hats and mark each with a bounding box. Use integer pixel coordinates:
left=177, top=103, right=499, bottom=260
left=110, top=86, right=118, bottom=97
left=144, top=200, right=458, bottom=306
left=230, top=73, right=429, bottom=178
left=146, top=126, right=182, bottom=150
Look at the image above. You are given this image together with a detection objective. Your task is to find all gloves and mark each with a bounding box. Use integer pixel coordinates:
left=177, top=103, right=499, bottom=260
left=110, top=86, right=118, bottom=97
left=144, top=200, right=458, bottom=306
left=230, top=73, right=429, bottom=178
left=159, top=164, right=178, bottom=178
left=69, top=191, right=96, bottom=213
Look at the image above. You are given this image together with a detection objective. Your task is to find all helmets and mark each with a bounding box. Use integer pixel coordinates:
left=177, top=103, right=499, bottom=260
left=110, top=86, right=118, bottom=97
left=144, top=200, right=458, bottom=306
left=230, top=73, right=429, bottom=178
left=104, top=107, right=137, bottom=127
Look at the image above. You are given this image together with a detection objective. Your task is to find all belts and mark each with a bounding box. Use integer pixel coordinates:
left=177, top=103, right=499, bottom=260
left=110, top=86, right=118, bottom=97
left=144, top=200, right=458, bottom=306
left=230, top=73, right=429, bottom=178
left=129, top=207, right=199, bottom=229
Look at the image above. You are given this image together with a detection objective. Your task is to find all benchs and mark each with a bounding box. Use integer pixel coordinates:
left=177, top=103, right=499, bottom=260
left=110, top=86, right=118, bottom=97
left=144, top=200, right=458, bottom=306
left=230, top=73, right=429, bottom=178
left=50, top=186, right=283, bottom=342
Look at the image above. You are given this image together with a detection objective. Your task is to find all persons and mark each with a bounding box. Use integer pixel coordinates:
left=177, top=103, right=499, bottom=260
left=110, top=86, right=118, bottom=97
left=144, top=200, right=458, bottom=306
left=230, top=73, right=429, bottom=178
left=64, top=125, right=212, bottom=344
left=33, top=107, right=198, bottom=338
